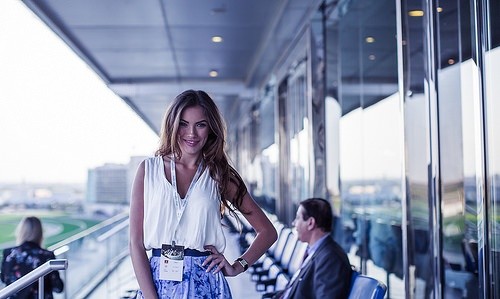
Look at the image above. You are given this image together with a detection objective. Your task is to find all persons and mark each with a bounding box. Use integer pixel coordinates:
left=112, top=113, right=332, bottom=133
left=0, top=217, right=64, bottom=299
left=277, top=198, right=352, bottom=299
left=131, top=89, right=278, bottom=299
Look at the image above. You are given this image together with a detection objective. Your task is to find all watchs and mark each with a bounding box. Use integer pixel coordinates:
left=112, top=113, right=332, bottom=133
left=236, top=258, right=249, bottom=272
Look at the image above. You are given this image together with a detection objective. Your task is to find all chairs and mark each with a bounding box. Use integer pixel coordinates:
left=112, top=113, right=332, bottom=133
left=237, top=213, right=387, bottom=299
